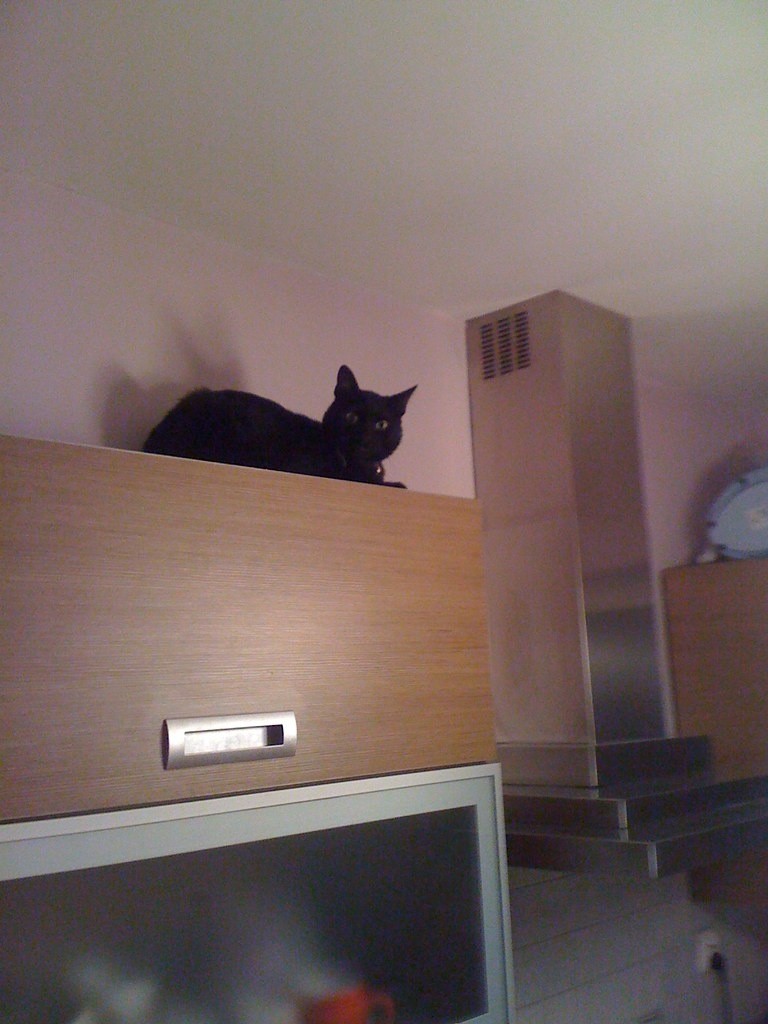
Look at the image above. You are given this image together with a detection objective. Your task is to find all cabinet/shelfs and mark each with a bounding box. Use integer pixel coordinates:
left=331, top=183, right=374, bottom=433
left=659, top=555, right=768, bottom=900
left=0, top=436, right=512, bottom=1024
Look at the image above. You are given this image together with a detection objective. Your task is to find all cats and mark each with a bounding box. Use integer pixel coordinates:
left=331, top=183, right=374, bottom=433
left=141, top=365, right=419, bottom=489
